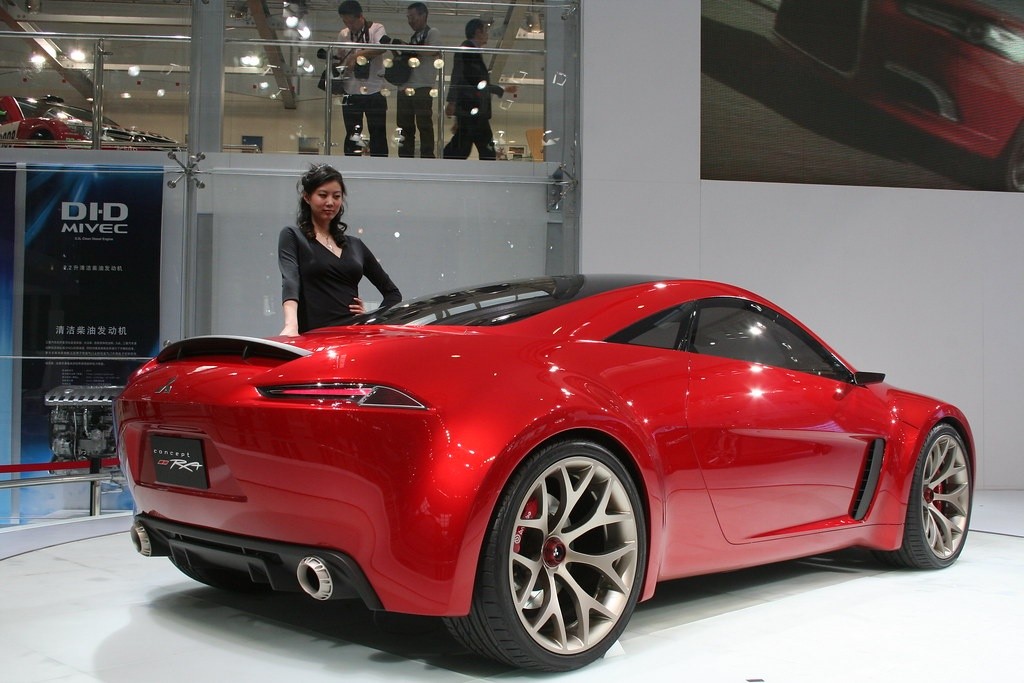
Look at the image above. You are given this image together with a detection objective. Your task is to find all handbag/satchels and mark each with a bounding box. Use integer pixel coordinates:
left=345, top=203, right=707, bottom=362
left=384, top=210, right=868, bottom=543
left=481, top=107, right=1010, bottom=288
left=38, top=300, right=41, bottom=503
left=385, top=53, right=416, bottom=84
left=318, top=63, right=342, bottom=94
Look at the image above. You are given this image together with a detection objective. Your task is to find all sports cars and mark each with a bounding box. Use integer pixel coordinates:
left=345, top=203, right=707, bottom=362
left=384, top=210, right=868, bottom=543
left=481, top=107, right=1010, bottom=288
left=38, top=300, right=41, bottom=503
left=0, top=95, right=188, bottom=152
left=115, top=274, right=976, bottom=673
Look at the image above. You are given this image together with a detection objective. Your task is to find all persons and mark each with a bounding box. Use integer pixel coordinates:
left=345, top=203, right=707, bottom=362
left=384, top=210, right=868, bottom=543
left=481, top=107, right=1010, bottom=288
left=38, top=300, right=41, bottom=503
left=444, top=18, right=519, bottom=160
left=278, top=163, right=402, bottom=339
left=335, top=0, right=388, bottom=157
left=391, top=2, right=443, bottom=157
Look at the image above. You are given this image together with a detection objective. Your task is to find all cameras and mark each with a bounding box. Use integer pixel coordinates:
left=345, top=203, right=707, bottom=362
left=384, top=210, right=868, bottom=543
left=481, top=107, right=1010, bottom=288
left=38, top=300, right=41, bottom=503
left=353, top=58, right=371, bottom=78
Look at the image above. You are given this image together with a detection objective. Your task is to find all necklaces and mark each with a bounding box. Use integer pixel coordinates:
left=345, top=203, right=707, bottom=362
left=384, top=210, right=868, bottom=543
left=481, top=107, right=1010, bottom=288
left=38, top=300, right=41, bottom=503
left=319, top=232, right=333, bottom=249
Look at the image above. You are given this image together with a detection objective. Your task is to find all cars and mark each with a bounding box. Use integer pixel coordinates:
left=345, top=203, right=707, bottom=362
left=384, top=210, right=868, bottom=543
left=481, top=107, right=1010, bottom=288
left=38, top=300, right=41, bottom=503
left=772, top=0, right=1024, bottom=193
